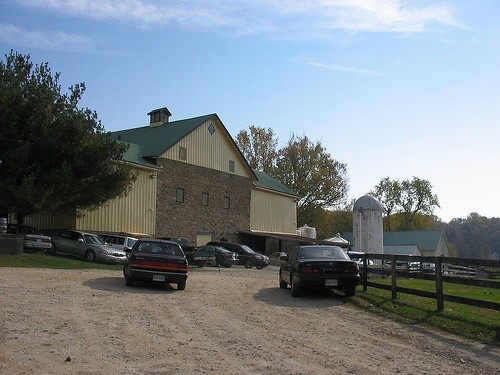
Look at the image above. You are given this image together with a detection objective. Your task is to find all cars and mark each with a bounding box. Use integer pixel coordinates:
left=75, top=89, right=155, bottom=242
left=280, top=246, right=360, bottom=297
left=7, top=223, right=52, bottom=253
left=347, top=251, right=374, bottom=265
left=190, top=245, right=239, bottom=266
left=123, top=239, right=188, bottom=291
left=47, top=229, right=126, bottom=263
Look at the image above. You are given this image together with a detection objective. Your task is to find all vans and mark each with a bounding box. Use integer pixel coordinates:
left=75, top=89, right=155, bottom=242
left=94, top=230, right=156, bottom=255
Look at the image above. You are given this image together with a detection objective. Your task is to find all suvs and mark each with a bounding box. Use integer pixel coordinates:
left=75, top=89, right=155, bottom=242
left=206, top=241, right=269, bottom=269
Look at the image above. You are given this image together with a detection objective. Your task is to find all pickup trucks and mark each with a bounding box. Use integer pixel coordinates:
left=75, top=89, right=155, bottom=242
left=158, top=236, right=216, bottom=267
